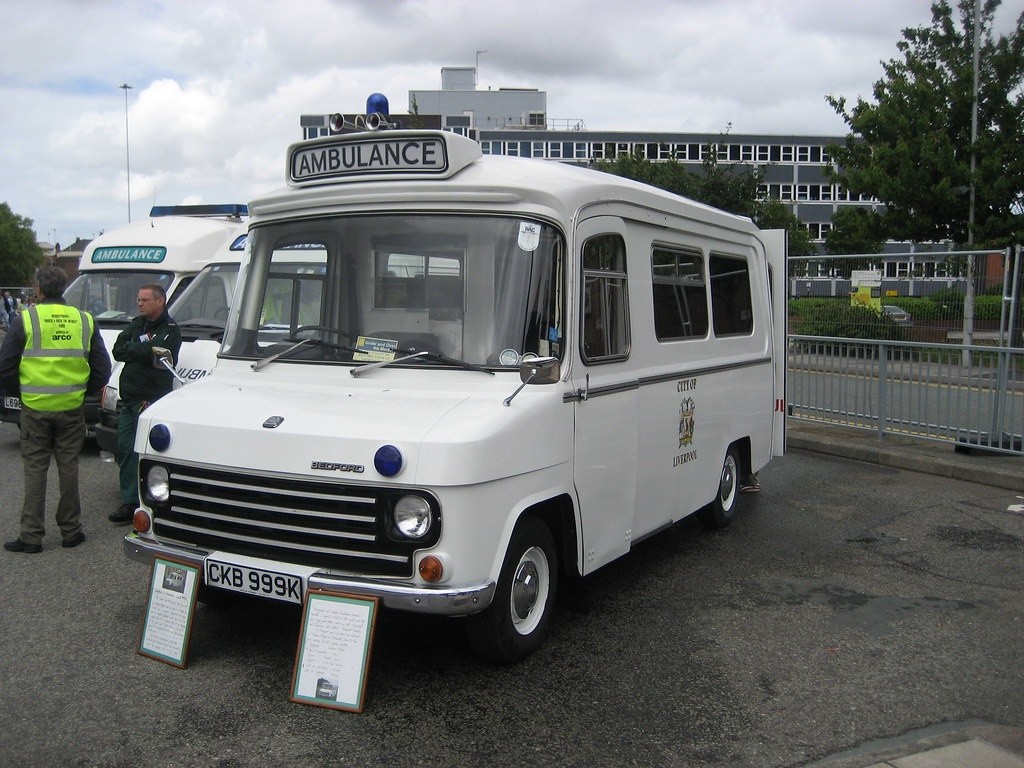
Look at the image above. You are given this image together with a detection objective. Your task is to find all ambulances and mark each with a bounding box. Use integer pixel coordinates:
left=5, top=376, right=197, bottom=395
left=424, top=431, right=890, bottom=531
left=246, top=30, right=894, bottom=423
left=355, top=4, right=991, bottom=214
left=92, top=222, right=460, bottom=466
left=0, top=205, right=250, bottom=435
left=124, top=95, right=790, bottom=659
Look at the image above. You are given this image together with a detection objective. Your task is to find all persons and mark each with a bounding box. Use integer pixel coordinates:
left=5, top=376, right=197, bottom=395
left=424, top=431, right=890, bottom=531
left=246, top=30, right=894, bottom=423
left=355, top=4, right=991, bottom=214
left=0, top=265, right=112, bottom=553
left=110, top=284, right=182, bottom=524
left=0, top=291, right=36, bottom=330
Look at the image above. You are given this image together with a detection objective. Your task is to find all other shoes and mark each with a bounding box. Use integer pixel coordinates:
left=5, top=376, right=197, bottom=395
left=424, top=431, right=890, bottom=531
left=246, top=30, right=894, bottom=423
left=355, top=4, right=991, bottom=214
left=5, top=541, right=42, bottom=553
left=109, top=504, right=140, bottom=522
left=62, top=533, right=84, bottom=547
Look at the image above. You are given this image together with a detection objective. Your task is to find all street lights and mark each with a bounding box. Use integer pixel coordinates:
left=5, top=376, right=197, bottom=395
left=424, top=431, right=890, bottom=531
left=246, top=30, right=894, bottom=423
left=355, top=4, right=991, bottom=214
left=117, top=79, right=136, bottom=225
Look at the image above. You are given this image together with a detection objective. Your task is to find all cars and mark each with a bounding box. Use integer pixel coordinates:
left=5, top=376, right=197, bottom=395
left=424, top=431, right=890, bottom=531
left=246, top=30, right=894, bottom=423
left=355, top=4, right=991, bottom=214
left=881, top=303, right=912, bottom=327
